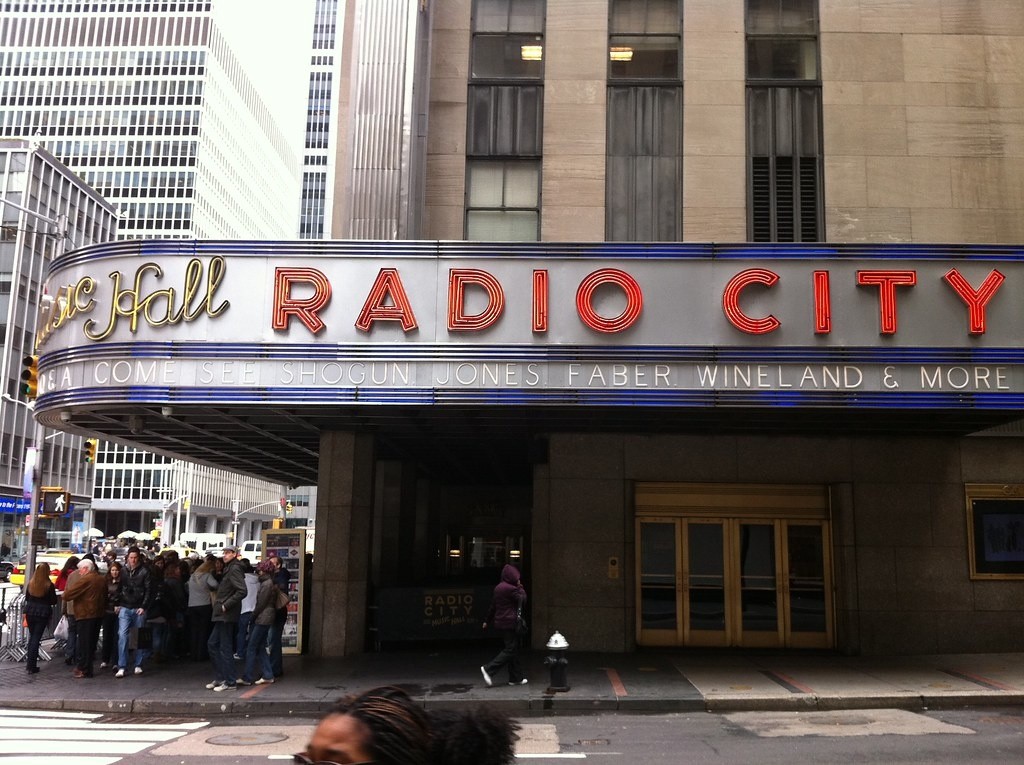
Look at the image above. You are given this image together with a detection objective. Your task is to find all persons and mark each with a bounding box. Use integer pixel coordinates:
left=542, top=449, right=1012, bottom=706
left=117, top=541, right=167, bottom=551
left=44, top=545, right=49, bottom=551
left=61, top=552, right=108, bottom=678
left=1, top=543, right=9, bottom=557
left=92, top=540, right=98, bottom=555
left=205, top=544, right=248, bottom=692
left=54, top=556, right=81, bottom=613
left=25, top=563, right=57, bottom=674
left=236, top=556, right=292, bottom=686
left=99, top=550, right=122, bottom=672
left=480, top=563, right=528, bottom=687
left=115, top=546, right=153, bottom=677
left=293, top=685, right=524, bottom=765
left=305, top=553, right=314, bottom=584
left=74, top=523, right=81, bottom=547
left=139, top=549, right=224, bottom=664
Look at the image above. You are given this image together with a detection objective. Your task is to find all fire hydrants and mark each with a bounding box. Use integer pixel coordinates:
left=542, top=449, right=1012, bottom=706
left=543, top=630, right=571, bottom=693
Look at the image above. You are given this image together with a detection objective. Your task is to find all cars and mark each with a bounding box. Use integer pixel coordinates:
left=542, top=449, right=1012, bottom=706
left=157, top=541, right=199, bottom=560
left=9, top=546, right=155, bottom=592
left=0, top=557, right=14, bottom=582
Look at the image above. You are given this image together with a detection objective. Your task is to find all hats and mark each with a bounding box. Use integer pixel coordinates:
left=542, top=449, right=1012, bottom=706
left=222, top=545, right=238, bottom=552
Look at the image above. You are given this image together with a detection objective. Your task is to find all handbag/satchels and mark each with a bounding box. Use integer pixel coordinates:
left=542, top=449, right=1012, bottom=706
left=515, top=616, right=528, bottom=636
left=53, top=614, right=69, bottom=640
left=274, top=587, right=291, bottom=609
left=209, top=590, right=217, bottom=608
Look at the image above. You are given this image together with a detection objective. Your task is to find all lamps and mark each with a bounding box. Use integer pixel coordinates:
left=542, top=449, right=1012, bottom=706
left=609, top=46, right=634, bottom=61
left=520, top=43, right=544, bottom=61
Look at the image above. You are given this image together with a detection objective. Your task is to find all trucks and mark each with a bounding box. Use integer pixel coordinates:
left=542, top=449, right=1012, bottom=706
left=295, top=526, right=315, bottom=555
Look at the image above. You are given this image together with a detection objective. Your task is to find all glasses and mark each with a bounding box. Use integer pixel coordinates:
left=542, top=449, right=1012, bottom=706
left=294, top=752, right=382, bottom=765
left=222, top=552, right=230, bottom=555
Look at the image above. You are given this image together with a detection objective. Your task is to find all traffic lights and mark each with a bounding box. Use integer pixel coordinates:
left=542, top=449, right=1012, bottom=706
left=83, top=440, right=96, bottom=464
left=20, top=354, right=40, bottom=399
left=286, top=505, right=292, bottom=514
left=44, top=491, right=71, bottom=515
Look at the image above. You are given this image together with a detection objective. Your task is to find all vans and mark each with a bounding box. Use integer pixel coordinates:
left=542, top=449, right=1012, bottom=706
left=178, top=532, right=226, bottom=558
left=239, top=540, right=263, bottom=568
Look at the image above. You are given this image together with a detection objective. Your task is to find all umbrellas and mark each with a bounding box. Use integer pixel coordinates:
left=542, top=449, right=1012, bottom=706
left=84, top=528, right=104, bottom=537
left=118, top=530, right=138, bottom=543
left=133, top=532, right=155, bottom=540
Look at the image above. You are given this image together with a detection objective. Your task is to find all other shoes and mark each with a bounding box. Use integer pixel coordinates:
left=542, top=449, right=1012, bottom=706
left=236, top=679, right=251, bottom=685
left=206, top=680, right=227, bottom=689
left=25, top=651, right=165, bottom=678
left=213, top=684, right=237, bottom=691
left=509, top=679, right=528, bottom=685
left=480, top=666, right=492, bottom=686
left=255, top=677, right=275, bottom=684
left=273, top=671, right=283, bottom=677
